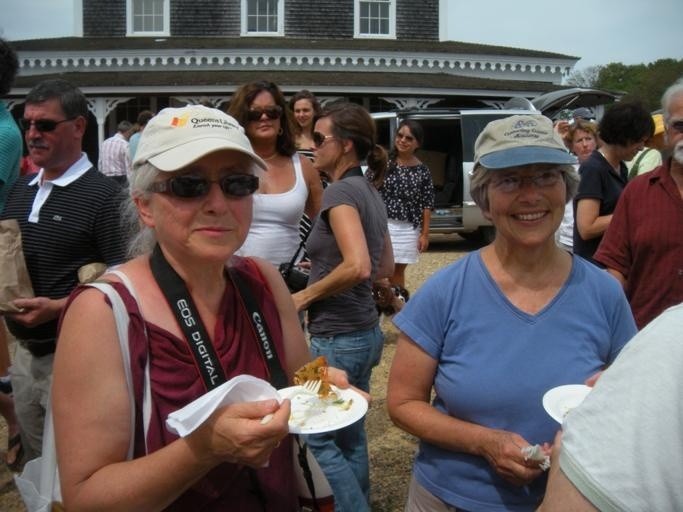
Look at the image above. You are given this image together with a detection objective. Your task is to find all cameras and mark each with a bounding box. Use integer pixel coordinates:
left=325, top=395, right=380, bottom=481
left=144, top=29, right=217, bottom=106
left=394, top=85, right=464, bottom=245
left=279, top=262, right=310, bottom=293
left=372, top=284, right=409, bottom=317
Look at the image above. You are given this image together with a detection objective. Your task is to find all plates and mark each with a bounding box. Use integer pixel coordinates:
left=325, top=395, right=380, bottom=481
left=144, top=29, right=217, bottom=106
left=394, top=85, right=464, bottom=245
left=265, top=383, right=369, bottom=438
left=541, top=383, right=593, bottom=426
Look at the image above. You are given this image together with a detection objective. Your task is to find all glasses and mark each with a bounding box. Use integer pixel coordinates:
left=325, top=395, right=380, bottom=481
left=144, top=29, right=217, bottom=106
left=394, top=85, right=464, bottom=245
left=246, top=106, right=282, bottom=121
left=19, top=117, right=71, bottom=132
left=147, top=174, right=259, bottom=200
left=490, top=170, right=560, bottom=192
left=311, top=131, right=337, bottom=149
left=397, top=132, right=414, bottom=143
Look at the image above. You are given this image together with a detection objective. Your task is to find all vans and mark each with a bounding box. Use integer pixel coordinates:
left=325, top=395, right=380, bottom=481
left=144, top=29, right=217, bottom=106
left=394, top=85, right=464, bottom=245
left=361, top=87, right=610, bottom=244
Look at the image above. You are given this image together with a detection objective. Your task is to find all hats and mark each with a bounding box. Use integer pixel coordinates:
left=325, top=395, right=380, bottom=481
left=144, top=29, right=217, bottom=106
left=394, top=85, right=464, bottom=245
left=573, top=108, right=596, bottom=120
left=473, top=115, right=576, bottom=170
left=132, top=104, right=270, bottom=174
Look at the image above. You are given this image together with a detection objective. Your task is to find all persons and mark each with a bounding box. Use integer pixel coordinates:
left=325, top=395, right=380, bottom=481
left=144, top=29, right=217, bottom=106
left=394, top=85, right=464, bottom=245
left=289, top=102, right=398, bottom=512
left=0, top=37, right=40, bottom=214
left=555, top=84, right=683, bottom=310
left=50, top=106, right=373, bottom=512
left=535, top=301, right=682, bottom=512
left=98, top=111, right=169, bottom=194
left=3, top=79, right=142, bottom=512
left=382, top=113, right=639, bottom=511
left=226, top=80, right=330, bottom=275
left=367, top=118, right=435, bottom=308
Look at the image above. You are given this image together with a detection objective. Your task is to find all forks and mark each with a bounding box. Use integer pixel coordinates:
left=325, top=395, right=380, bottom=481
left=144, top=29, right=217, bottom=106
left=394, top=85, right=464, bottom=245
left=262, top=378, right=323, bottom=426
